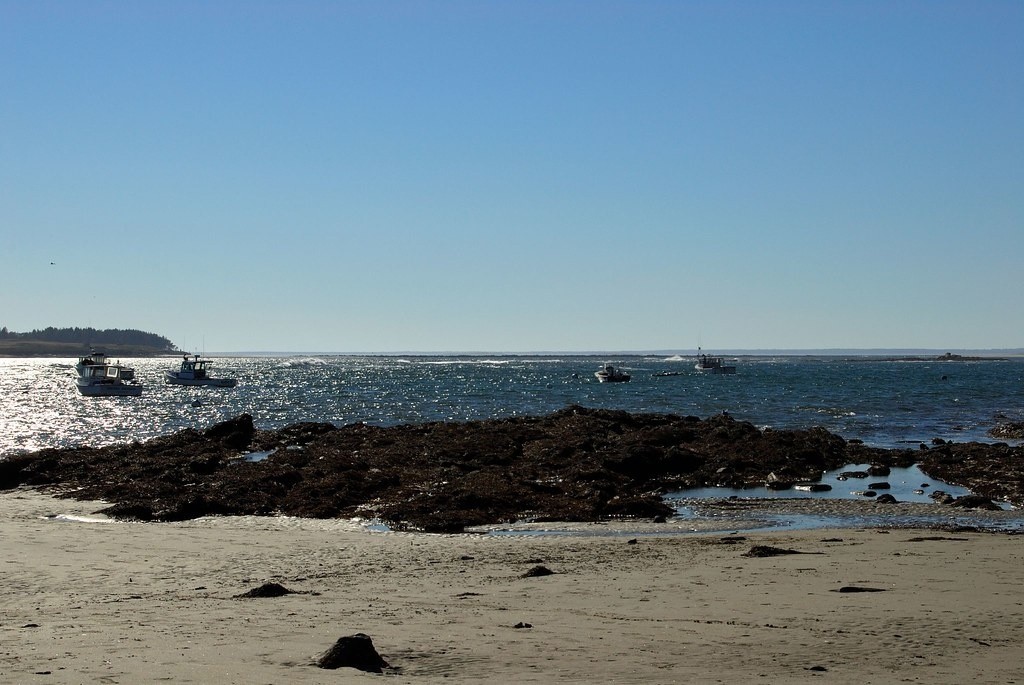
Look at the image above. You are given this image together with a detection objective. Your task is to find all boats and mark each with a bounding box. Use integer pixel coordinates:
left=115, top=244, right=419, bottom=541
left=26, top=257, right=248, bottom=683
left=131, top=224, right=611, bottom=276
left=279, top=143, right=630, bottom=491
left=74, top=346, right=142, bottom=397
left=695, top=356, right=736, bottom=374
left=594, top=363, right=631, bottom=383
left=163, top=353, right=237, bottom=388
